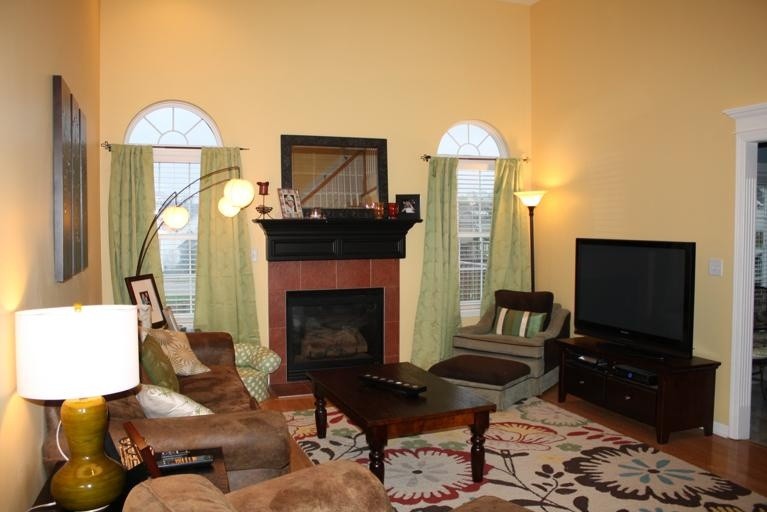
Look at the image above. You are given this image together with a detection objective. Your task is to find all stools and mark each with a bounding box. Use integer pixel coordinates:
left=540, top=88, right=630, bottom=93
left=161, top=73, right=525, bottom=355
left=429, top=354, right=531, bottom=411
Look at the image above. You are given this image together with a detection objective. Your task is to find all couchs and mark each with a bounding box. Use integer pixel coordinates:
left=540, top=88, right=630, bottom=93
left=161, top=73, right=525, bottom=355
left=42, top=331, right=291, bottom=494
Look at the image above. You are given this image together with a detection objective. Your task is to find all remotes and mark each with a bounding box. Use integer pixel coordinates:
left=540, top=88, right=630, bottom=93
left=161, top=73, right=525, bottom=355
left=157, top=455, right=214, bottom=468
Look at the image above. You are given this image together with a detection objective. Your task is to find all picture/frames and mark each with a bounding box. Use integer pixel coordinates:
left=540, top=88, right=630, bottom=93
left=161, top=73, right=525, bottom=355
left=396, top=194, right=420, bottom=218
left=123, top=421, right=161, bottom=478
left=124, top=273, right=168, bottom=328
left=277, top=188, right=304, bottom=219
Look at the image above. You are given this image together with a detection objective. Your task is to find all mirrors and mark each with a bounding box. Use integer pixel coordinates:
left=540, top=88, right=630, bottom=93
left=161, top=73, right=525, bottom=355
left=280, top=134, right=388, bottom=221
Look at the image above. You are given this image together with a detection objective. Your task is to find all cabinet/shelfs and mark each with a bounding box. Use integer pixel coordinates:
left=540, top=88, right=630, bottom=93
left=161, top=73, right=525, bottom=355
left=558, top=348, right=717, bottom=445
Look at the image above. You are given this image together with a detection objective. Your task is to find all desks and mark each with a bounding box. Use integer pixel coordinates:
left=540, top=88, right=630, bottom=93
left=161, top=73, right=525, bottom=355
left=28, top=447, right=230, bottom=512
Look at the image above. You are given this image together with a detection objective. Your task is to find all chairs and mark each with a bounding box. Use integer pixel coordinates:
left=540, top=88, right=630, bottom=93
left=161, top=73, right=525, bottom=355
left=233, top=343, right=282, bottom=404
left=752, top=329, right=767, bottom=401
left=452, top=303, right=571, bottom=397
left=121, top=458, right=536, bottom=512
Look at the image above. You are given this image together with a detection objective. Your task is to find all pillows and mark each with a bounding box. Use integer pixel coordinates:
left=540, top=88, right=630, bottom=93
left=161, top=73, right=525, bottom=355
left=492, top=289, right=554, bottom=331
left=136, top=331, right=215, bottom=420
left=492, top=307, right=547, bottom=338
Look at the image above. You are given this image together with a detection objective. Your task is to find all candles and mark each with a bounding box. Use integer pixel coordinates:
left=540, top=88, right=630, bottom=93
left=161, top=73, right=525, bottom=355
left=256, top=181, right=270, bottom=195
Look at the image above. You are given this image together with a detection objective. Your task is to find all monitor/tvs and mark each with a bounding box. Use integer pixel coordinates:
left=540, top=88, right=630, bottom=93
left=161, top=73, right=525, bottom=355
left=573, top=238, right=696, bottom=363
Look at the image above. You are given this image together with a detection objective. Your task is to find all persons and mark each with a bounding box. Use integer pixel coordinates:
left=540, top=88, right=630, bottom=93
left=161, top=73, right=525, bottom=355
left=140, top=292, right=150, bottom=303
left=284, top=195, right=295, bottom=212
left=401, top=200, right=416, bottom=213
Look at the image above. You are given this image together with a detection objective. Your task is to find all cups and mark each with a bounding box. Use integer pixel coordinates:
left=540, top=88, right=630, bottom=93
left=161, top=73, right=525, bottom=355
left=374, top=201, right=385, bottom=220
left=385, top=203, right=399, bottom=220
left=309, top=207, right=321, bottom=219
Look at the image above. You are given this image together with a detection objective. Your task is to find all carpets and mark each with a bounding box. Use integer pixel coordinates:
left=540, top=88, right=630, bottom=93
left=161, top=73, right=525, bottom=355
left=279, top=396, right=766, bottom=512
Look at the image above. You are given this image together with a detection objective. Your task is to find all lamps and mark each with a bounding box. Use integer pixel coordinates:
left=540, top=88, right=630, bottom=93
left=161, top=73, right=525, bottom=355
left=14, top=304, right=140, bottom=512
left=134, top=165, right=255, bottom=275
left=512, top=190, right=549, bottom=292
